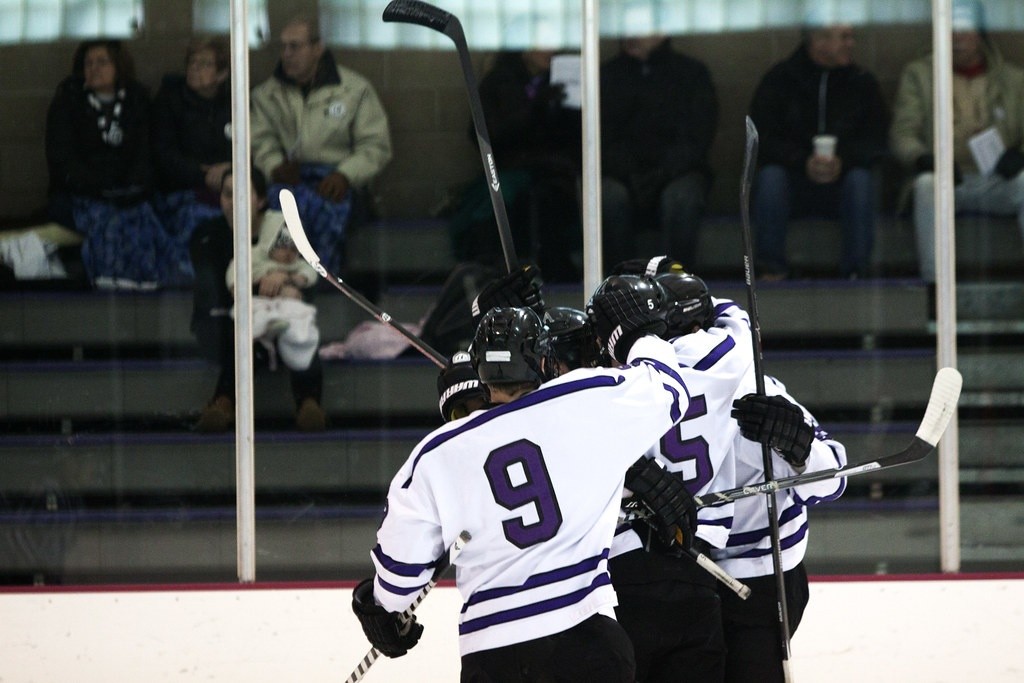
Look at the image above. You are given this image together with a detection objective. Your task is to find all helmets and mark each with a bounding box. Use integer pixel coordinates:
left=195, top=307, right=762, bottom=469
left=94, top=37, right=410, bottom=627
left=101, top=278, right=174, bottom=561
left=654, top=272, right=716, bottom=340
left=593, top=274, right=669, bottom=337
left=470, top=307, right=589, bottom=385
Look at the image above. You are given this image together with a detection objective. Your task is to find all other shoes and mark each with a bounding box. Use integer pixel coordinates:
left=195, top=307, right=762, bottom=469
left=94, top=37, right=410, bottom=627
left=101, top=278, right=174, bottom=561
left=296, top=398, right=326, bottom=433
left=196, top=396, right=235, bottom=433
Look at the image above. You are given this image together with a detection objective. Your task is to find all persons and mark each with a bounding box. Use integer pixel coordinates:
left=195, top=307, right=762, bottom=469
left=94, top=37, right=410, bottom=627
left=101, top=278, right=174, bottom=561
left=45, top=15, right=392, bottom=291
left=750, top=0, right=890, bottom=284
left=190, top=166, right=328, bottom=432
left=449, top=0, right=721, bottom=274
left=888, top=0, right=1024, bottom=320
left=352, top=255, right=848, bottom=683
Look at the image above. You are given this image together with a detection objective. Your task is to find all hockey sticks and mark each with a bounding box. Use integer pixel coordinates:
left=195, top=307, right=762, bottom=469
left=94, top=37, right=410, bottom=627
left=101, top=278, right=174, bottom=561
left=274, top=184, right=449, bottom=371
left=380, top=0, right=521, bottom=278
left=619, top=367, right=965, bottom=534
left=341, top=528, right=474, bottom=683
left=739, top=111, right=792, bottom=665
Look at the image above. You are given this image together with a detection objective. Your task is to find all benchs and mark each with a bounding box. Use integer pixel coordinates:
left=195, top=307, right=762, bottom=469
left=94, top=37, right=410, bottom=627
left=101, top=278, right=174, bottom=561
left=0, top=203, right=1024, bottom=574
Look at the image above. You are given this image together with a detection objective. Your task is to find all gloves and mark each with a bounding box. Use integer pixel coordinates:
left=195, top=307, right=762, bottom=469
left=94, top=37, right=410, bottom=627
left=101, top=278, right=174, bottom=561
left=317, top=173, right=351, bottom=201
left=272, top=163, right=301, bottom=184
left=471, top=266, right=545, bottom=327
left=590, top=289, right=667, bottom=364
left=626, top=458, right=699, bottom=554
left=436, top=361, right=487, bottom=418
left=710, top=395, right=816, bottom=467
left=350, top=577, right=424, bottom=659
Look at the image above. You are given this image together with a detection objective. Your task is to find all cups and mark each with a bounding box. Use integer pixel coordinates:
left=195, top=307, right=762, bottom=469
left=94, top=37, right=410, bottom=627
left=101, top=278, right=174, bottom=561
left=812, top=134, right=838, bottom=162
